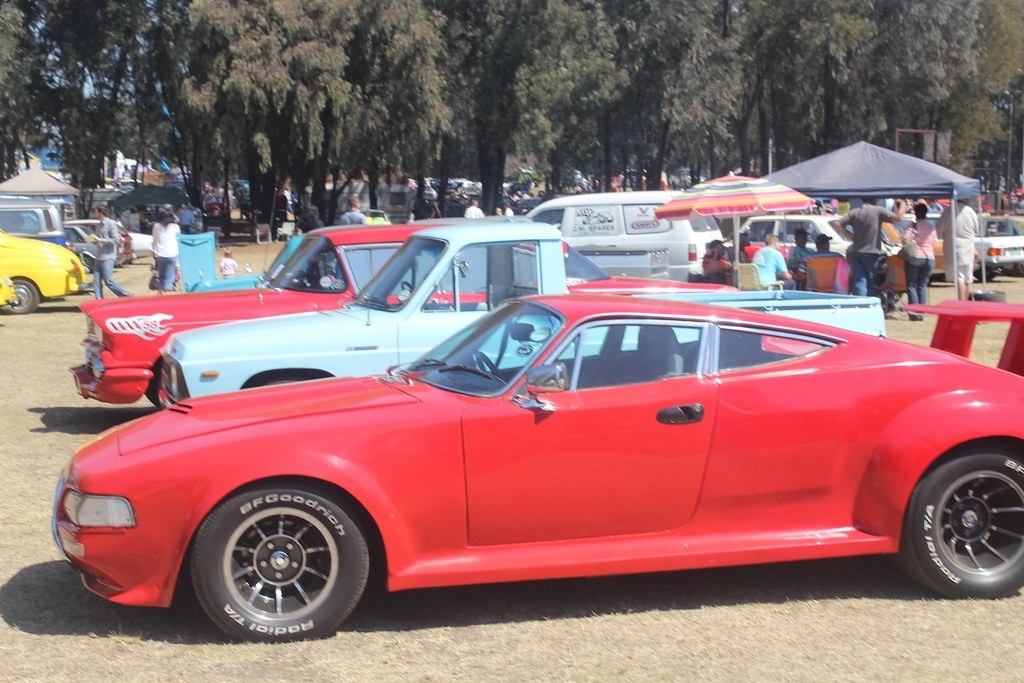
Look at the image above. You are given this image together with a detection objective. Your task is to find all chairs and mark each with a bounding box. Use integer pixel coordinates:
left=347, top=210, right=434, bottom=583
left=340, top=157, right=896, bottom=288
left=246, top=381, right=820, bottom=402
left=208, top=225, right=222, bottom=248
left=636, top=324, right=682, bottom=382
left=276, top=220, right=295, bottom=243
left=738, top=262, right=785, bottom=291
left=254, top=223, right=273, bottom=244
left=806, top=256, right=842, bottom=294
left=875, top=253, right=910, bottom=320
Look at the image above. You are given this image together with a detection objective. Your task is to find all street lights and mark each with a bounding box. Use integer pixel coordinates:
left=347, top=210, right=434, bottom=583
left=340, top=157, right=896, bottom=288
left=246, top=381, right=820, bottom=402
left=1003, top=88, right=1023, bottom=194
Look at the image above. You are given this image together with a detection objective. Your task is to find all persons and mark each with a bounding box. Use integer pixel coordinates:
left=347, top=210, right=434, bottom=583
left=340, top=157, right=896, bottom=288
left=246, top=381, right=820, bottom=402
left=903, top=204, right=937, bottom=320
left=296, top=206, right=322, bottom=234
left=495, top=190, right=534, bottom=216
left=815, top=197, right=943, bottom=214
left=705, top=228, right=844, bottom=289
left=277, top=189, right=288, bottom=220
left=84, top=204, right=133, bottom=299
left=128, top=204, right=199, bottom=235
left=149, top=208, right=181, bottom=293
left=201, top=188, right=250, bottom=220
left=937, top=200, right=980, bottom=300
left=219, top=249, right=238, bottom=277
left=340, top=197, right=366, bottom=224
left=834, top=198, right=906, bottom=297
left=464, top=198, right=483, bottom=217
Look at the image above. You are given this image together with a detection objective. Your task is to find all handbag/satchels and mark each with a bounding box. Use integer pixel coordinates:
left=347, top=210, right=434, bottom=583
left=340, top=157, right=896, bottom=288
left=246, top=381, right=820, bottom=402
left=897, top=222, right=916, bottom=260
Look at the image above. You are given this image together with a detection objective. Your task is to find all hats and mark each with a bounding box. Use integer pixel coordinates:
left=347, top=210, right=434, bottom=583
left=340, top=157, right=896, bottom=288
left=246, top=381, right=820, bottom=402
left=795, top=228, right=810, bottom=234
left=815, top=234, right=832, bottom=244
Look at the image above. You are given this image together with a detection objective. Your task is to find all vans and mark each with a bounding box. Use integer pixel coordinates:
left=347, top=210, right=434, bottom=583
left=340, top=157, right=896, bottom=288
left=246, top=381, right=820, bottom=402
left=523, top=192, right=723, bottom=284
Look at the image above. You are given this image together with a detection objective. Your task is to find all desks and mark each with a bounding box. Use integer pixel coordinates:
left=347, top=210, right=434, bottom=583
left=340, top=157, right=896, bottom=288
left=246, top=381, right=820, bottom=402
left=230, top=232, right=252, bottom=248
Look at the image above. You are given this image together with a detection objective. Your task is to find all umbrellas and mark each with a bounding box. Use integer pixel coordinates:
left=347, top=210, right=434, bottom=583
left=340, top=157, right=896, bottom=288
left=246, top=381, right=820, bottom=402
left=655, top=170, right=818, bottom=285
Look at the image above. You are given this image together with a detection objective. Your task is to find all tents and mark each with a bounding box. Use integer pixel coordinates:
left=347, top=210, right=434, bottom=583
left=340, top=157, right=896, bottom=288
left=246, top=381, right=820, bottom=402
left=737, top=140, right=986, bottom=299
left=0, top=166, right=84, bottom=219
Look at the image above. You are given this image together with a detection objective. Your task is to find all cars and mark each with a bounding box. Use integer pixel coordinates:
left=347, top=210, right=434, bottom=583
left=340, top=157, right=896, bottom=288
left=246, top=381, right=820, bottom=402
left=738, top=214, right=1024, bottom=282
left=0, top=199, right=153, bottom=315
left=670, top=167, right=706, bottom=188
left=623, top=169, right=648, bottom=187
left=52, top=295, right=1024, bottom=642
left=69, top=170, right=614, bottom=404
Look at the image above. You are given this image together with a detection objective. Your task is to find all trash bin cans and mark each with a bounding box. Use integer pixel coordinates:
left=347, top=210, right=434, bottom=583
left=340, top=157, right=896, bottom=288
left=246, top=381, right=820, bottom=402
left=203, top=213, right=223, bottom=242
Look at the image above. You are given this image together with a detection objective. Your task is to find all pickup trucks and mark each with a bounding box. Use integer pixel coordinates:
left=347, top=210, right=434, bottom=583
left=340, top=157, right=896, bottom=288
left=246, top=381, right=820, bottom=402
left=155, top=221, right=886, bottom=409
